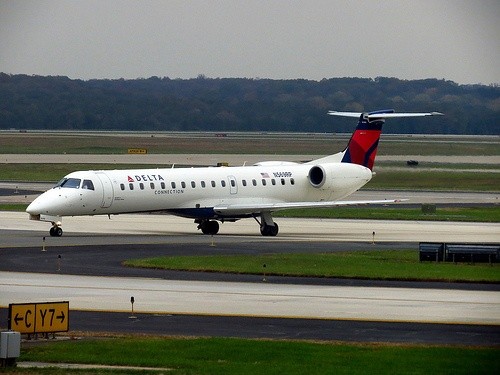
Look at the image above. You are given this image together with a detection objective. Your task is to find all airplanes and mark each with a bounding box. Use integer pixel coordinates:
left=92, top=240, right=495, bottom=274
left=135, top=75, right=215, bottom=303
left=26, top=109, right=446, bottom=236
